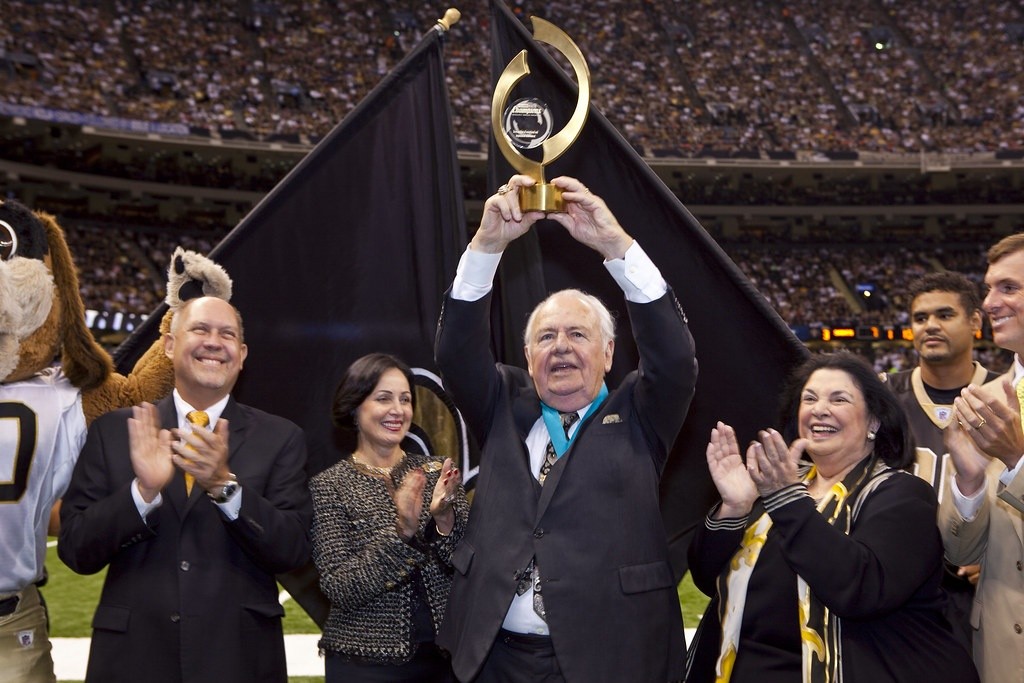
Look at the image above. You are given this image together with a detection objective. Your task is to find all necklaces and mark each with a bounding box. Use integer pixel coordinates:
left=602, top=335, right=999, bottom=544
left=352, top=453, right=408, bottom=474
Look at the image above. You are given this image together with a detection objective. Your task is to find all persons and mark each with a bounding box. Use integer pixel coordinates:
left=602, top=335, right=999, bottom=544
left=310, top=351, right=472, bottom=683
left=938, top=233, right=1024, bottom=683
left=434, top=176, right=700, bottom=683
left=877, top=268, right=1004, bottom=650
left=0, top=0, right=1024, bottom=376
left=686, top=348, right=983, bottom=683
left=57, top=296, right=314, bottom=683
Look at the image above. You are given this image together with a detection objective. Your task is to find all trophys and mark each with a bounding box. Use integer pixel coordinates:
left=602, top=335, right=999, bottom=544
left=490, top=16, right=590, bottom=215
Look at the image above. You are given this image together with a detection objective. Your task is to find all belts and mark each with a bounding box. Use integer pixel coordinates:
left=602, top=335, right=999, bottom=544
left=0, top=597, right=18, bottom=616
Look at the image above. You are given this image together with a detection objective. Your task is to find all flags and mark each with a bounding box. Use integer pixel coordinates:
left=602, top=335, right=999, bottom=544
left=484, top=0, right=815, bottom=596
left=111, top=25, right=482, bottom=634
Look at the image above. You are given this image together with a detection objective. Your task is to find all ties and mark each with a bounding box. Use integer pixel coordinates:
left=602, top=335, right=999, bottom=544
left=515, top=412, right=579, bottom=597
left=182, top=410, right=209, bottom=498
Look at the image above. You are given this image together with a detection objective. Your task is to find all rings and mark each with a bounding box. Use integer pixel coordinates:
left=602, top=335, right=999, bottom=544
left=585, top=188, right=589, bottom=194
left=976, top=422, right=984, bottom=429
left=446, top=496, right=455, bottom=503
left=499, top=184, right=512, bottom=195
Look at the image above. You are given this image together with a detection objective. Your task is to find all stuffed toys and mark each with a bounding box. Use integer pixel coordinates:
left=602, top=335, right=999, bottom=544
left=0, top=202, right=231, bottom=683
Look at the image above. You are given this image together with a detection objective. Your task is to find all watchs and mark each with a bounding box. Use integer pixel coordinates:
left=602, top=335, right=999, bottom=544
left=207, top=473, right=241, bottom=503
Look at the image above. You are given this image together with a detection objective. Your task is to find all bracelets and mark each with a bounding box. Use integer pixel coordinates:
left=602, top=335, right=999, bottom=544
left=436, top=526, right=451, bottom=537
